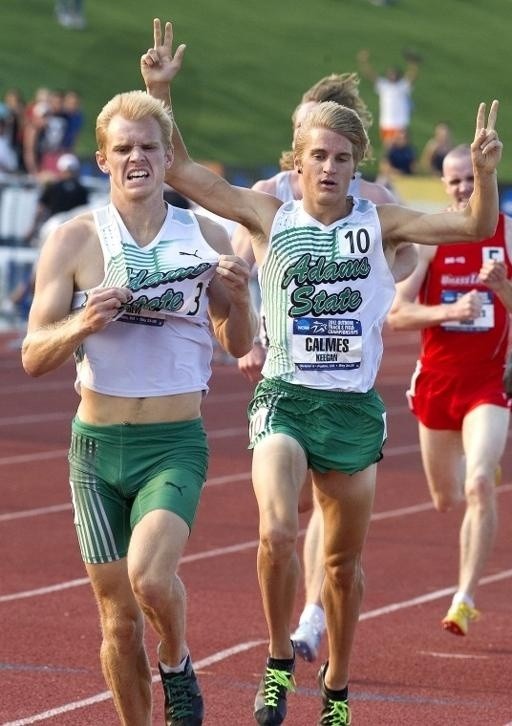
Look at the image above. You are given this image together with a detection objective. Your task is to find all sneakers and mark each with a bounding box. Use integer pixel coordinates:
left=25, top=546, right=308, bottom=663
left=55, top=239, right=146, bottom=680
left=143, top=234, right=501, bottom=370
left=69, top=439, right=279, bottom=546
left=441, top=601, right=480, bottom=637
left=253, top=623, right=351, bottom=725
left=158, top=653, right=203, bottom=726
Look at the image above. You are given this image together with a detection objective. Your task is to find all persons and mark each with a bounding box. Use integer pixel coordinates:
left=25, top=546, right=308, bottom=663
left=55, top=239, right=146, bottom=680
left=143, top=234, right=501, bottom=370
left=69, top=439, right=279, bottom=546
left=384, top=140, right=511, bottom=638
left=17, top=88, right=264, bottom=726
left=216, top=71, right=419, bottom=664
left=50, top=0, right=90, bottom=31
left=353, top=47, right=418, bottom=154
left=418, top=120, right=454, bottom=206
left=382, top=143, right=427, bottom=202
left=137, top=16, right=503, bottom=726
left=0, top=85, right=92, bottom=354
